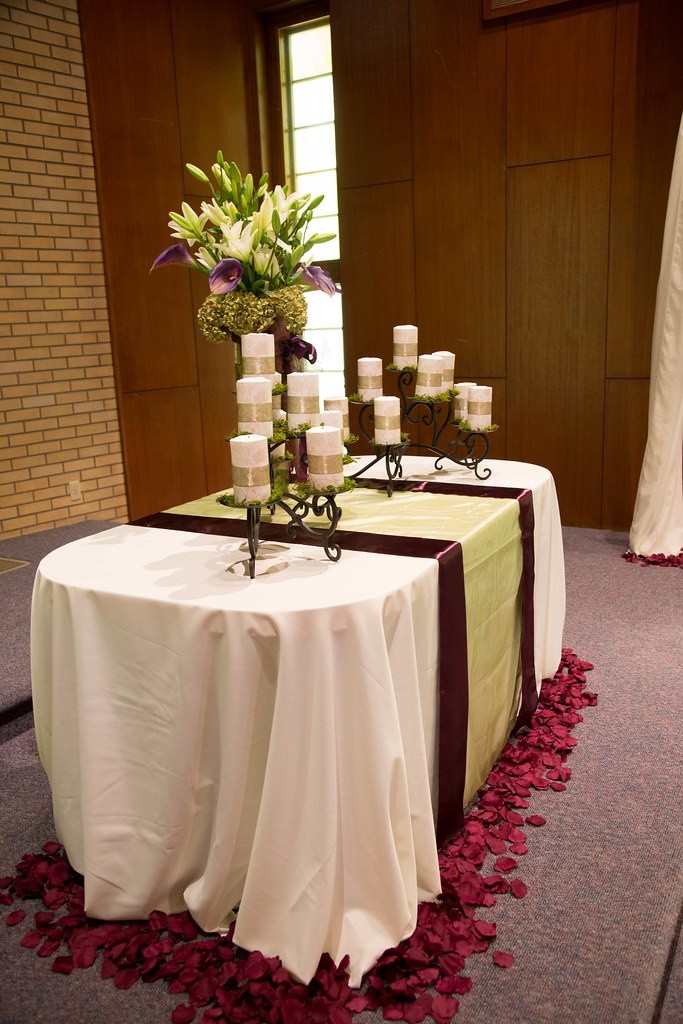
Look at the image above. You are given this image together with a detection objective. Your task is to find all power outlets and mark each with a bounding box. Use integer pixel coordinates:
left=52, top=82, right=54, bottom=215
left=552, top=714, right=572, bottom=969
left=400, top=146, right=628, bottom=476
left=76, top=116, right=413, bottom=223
left=68, top=480, right=82, bottom=501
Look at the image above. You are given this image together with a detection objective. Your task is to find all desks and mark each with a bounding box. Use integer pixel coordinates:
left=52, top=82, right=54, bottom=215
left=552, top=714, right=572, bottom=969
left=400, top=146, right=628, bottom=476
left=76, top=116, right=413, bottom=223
left=30, top=456, right=566, bottom=987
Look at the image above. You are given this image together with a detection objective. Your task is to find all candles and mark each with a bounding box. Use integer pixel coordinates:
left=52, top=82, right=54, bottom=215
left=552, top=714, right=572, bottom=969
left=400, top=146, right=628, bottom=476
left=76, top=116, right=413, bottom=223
left=393, top=324, right=418, bottom=370
left=316, top=411, right=348, bottom=457
left=415, top=353, right=444, bottom=396
left=323, top=397, right=349, bottom=440
left=230, top=434, right=271, bottom=503
left=286, top=372, right=319, bottom=430
left=433, top=350, right=455, bottom=392
left=468, top=386, right=493, bottom=429
left=307, top=425, right=344, bottom=489
left=452, top=381, right=477, bottom=420
left=357, top=357, right=384, bottom=402
left=374, top=396, right=401, bottom=445
left=236, top=332, right=286, bottom=458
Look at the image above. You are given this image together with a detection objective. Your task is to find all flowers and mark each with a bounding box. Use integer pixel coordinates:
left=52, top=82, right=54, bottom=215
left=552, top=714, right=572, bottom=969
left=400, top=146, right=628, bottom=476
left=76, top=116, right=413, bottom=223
left=149, top=149, right=346, bottom=297
